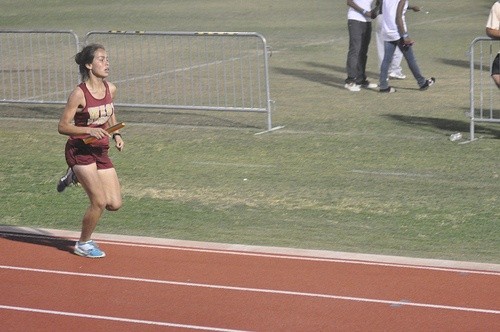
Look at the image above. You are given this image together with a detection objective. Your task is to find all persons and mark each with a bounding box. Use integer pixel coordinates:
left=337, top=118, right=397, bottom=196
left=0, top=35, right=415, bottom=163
left=378, top=0, right=436, bottom=94
left=486, top=0, right=500, bottom=90
left=56, top=43, right=125, bottom=258
left=375, top=14, right=407, bottom=80
left=344, top=0, right=382, bottom=91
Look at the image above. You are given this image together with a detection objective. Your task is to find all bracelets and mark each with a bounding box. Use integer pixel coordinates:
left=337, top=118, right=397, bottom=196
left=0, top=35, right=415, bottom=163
left=401, top=33, right=408, bottom=38
left=113, top=133, right=122, bottom=140
left=362, top=9, right=367, bottom=15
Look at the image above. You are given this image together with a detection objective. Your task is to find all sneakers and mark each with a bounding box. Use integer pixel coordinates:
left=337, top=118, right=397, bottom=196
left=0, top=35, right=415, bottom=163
left=362, top=82, right=377, bottom=88
left=344, top=82, right=360, bottom=91
left=380, top=87, right=397, bottom=93
left=389, top=72, right=406, bottom=79
left=57, top=167, right=78, bottom=192
left=421, top=77, right=436, bottom=91
left=74, top=240, right=105, bottom=257
